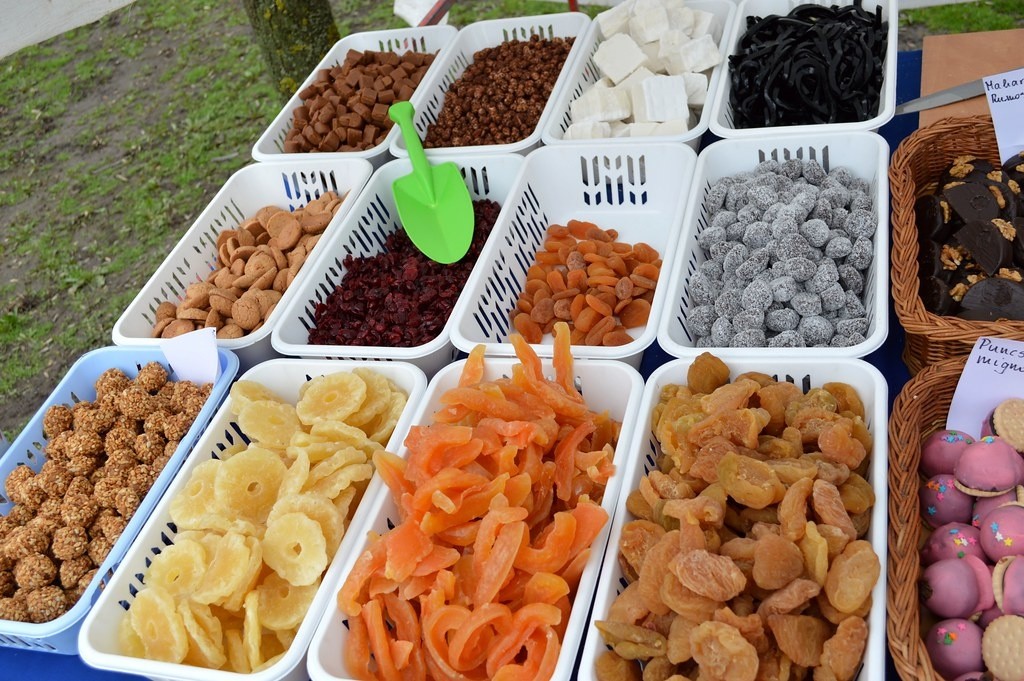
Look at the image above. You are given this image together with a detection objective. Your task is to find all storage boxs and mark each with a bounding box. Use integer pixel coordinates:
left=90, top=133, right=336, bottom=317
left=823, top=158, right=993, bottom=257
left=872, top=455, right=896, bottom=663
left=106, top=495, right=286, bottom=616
left=390, top=11, right=590, bottom=153
left=80, top=357, right=427, bottom=681
left=541, top=0, right=736, bottom=145
left=272, top=153, right=524, bottom=357
left=451, top=144, right=697, bottom=359
left=709, top=0, right=899, bottom=138
left=0, top=344, right=239, bottom=656
left=256, top=25, right=458, bottom=166
left=307, top=359, right=644, bottom=681
left=113, top=157, right=374, bottom=345
left=660, top=134, right=888, bottom=357
left=581, top=356, right=888, bottom=681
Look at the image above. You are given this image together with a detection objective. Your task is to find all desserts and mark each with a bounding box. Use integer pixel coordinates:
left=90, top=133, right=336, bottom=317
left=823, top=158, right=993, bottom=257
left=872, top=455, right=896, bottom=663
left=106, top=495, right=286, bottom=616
left=911, top=153, right=1024, bottom=681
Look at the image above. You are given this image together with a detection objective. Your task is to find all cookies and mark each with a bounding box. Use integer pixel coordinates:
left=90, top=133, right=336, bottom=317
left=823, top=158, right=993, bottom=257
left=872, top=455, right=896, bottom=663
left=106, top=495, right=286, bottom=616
left=151, top=192, right=351, bottom=343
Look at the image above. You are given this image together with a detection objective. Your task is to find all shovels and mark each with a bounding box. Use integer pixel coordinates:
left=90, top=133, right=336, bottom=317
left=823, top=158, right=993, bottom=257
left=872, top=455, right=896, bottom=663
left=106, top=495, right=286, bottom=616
left=386, top=100, right=475, bottom=265
